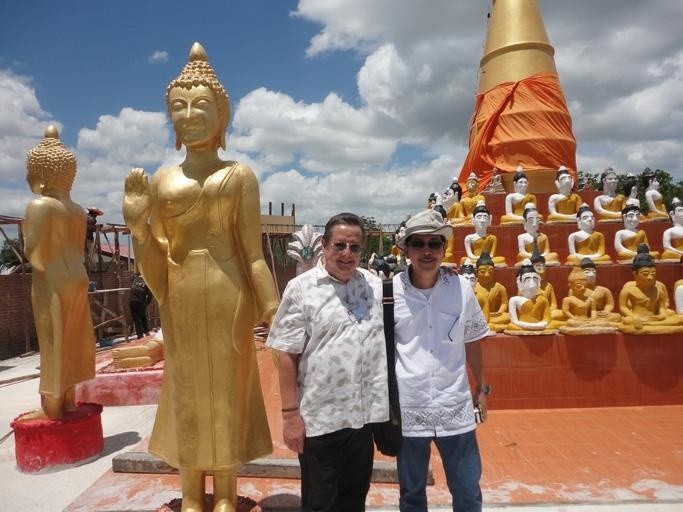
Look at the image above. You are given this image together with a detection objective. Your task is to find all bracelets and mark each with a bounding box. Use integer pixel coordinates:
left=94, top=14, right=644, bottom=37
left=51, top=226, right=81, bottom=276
left=282, top=407, right=298, bottom=412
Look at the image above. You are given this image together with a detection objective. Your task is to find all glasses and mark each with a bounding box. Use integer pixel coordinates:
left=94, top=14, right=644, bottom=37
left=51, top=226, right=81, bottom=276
left=406, top=239, right=445, bottom=248
left=327, top=241, right=364, bottom=254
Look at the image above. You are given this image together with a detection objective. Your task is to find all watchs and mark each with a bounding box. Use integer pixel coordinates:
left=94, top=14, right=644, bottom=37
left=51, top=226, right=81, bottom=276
left=476, top=384, right=493, bottom=396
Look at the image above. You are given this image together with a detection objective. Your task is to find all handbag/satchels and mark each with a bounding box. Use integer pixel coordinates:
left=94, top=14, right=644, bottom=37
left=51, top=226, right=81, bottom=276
left=375, top=365, right=402, bottom=457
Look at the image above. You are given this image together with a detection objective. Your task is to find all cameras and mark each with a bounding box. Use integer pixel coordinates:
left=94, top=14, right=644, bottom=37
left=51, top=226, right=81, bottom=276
left=473, top=406, right=483, bottom=424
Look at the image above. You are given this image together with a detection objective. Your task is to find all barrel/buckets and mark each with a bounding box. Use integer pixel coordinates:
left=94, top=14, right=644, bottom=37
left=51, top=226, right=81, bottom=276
left=100, top=337, right=113, bottom=349
left=89, top=282, right=98, bottom=294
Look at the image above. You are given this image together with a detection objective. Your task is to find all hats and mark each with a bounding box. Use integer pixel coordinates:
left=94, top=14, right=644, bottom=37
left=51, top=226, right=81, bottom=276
left=394, top=209, right=455, bottom=251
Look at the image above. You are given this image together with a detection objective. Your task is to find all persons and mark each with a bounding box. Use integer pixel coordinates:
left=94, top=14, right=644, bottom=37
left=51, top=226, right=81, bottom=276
left=129, top=274, right=154, bottom=338
left=267, top=213, right=389, bottom=511
left=122, top=42, right=281, bottom=512
left=19, top=125, right=96, bottom=421
left=383, top=210, right=492, bottom=511
left=392, top=165, right=682, bottom=335
